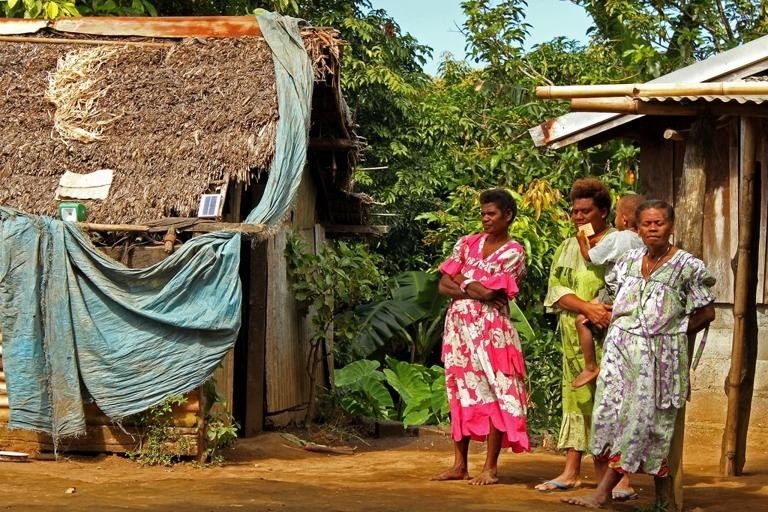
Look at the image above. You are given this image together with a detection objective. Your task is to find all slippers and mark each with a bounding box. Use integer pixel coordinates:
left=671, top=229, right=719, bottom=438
left=611, top=490, right=638, bottom=501
left=534, top=481, right=567, bottom=493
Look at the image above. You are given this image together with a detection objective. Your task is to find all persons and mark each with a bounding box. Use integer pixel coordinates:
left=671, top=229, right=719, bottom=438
left=430, top=188, right=532, bottom=484
left=559, top=199, right=715, bottom=511
left=534, top=177, right=640, bottom=502
left=571, top=196, right=648, bottom=387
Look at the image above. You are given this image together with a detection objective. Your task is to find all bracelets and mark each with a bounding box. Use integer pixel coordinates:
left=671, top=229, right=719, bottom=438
left=460, top=279, right=477, bottom=293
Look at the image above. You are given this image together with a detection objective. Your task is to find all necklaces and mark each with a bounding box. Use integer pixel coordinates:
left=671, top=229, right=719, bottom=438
left=646, top=246, right=669, bottom=275
